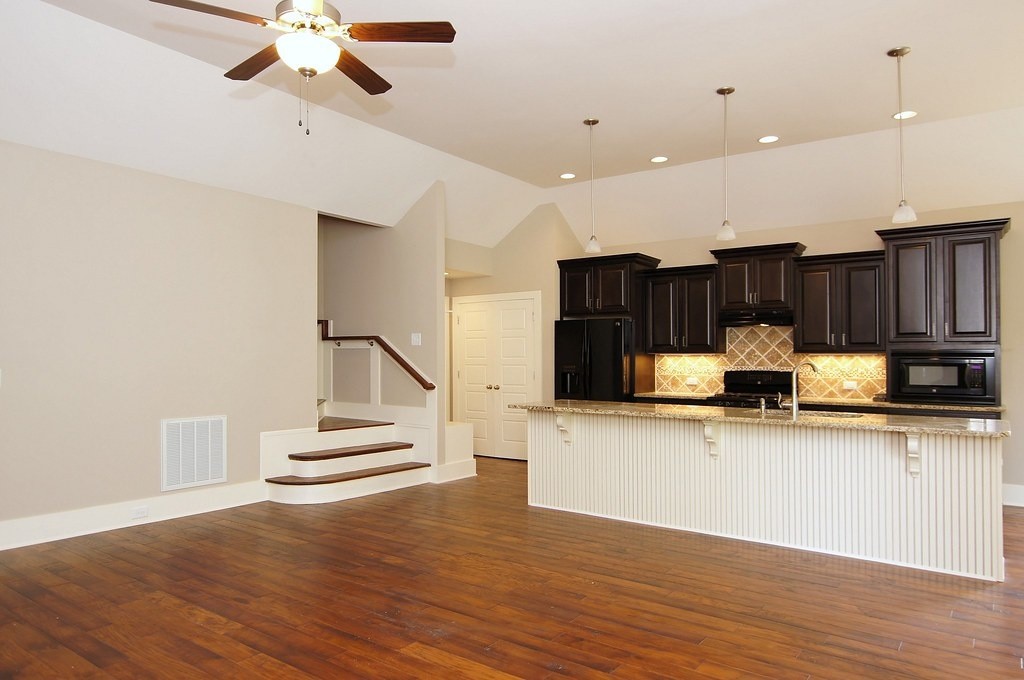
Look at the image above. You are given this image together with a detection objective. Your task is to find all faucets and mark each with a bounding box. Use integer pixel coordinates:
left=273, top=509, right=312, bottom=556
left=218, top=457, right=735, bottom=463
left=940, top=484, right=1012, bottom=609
left=791, top=361, right=819, bottom=421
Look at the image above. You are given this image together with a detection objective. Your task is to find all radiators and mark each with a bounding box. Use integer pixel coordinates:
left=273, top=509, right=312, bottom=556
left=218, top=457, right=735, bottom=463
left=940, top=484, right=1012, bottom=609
left=160, top=416, right=228, bottom=493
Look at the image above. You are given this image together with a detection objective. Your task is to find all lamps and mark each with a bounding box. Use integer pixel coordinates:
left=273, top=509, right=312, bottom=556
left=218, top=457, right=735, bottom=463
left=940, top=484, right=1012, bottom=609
left=887, top=46, right=917, bottom=223
left=275, top=23, right=342, bottom=136
left=582, top=118, right=601, bottom=253
left=716, top=86, right=737, bottom=241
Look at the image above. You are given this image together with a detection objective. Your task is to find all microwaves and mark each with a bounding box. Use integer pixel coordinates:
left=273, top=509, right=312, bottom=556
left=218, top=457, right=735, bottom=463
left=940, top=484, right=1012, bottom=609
left=895, top=357, right=995, bottom=401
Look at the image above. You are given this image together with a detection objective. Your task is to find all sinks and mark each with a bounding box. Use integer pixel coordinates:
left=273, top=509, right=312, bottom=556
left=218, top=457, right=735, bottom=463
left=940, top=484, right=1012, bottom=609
left=776, top=409, right=865, bottom=423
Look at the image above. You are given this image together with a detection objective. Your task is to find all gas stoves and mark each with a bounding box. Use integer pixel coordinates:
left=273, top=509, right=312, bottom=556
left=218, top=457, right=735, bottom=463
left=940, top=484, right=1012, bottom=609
left=706, top=393, right=793, bottom=408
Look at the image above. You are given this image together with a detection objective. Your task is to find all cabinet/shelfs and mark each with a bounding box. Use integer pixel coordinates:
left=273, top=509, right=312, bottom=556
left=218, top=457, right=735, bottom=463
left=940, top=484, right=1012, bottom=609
left=793, top=249, right=885, bottom=355
left=709, top=242, right=808, bottom=325
left=635, top=263, right=727, bottom=355
left=553, top=252, right=662, bottom=402
left=876, top=216, right=1012, bottom=406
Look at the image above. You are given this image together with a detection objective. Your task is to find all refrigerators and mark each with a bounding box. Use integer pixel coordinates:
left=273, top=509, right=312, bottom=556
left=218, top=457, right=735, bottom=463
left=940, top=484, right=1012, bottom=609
left=554, top=318, right=634, bottom=402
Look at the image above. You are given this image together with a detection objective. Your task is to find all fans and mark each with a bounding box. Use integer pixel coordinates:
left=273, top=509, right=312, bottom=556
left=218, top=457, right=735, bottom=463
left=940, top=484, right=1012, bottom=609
left=150, top=0, right=456, bottom=97
